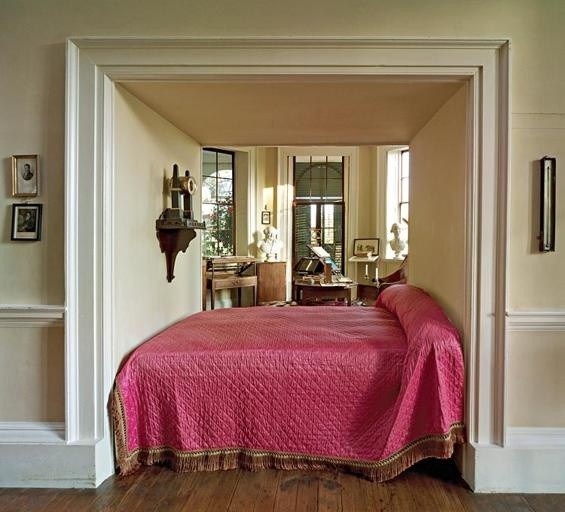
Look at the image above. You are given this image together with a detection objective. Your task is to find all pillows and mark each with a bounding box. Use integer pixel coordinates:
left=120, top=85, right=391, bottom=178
left=373, top=284, right=462, bottom=357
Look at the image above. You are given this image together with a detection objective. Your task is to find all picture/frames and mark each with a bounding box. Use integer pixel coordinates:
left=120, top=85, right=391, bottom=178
left=353, top=238, right=379, bottom=256
left=11, top=203, right=43, bottom=241
left=12, top=154, right=40, bottom=197
left=262, top=211, right=270, bottom=224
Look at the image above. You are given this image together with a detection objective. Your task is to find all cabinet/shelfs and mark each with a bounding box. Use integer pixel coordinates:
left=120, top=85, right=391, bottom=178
left=202, top=256, right=258, bottom=311
left=252, top=262, right=287, bottom=305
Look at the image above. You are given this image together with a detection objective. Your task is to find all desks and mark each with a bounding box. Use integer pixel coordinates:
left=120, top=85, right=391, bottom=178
left=292, top=285, right=358, bottom=306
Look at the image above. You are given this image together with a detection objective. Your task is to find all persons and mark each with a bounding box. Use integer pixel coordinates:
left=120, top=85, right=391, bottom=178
left=19, top=212, right=34, bottom=231
left=22, top=164, right=33, bottom=180
left=389, top=223, right=406, bottom=250
left=257, top=226, right=284, bottom=253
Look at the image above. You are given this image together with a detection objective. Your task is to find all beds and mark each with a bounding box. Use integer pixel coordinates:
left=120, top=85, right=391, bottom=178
left=108, top=284, right=466, bottom=482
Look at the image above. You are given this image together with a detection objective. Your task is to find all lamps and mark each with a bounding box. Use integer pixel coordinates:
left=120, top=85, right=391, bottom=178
left=538, top=156, right=555, bottom=253
